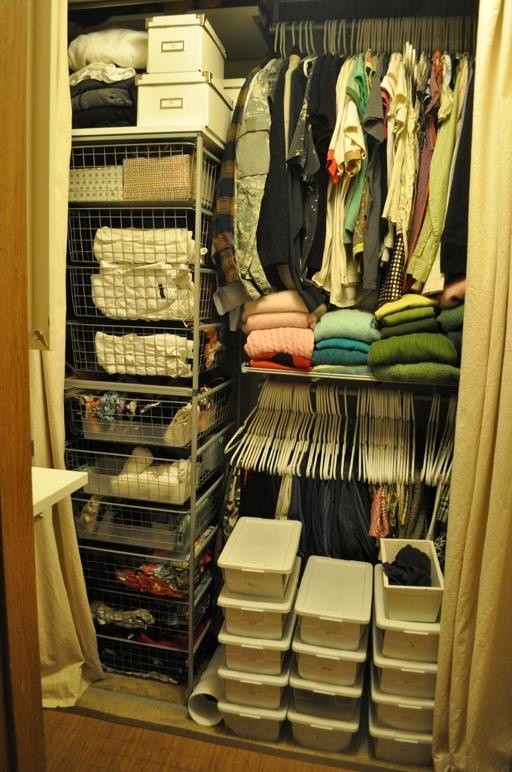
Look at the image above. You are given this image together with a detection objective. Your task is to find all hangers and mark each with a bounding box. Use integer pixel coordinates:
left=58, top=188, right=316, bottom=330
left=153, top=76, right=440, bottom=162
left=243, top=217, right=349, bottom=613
left=262, top=14, right=477, bottom=62
left=220, top=371, right=458, bottom=489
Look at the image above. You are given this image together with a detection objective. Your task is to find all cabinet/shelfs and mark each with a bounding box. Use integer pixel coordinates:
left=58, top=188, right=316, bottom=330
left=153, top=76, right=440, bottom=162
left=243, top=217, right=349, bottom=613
left=61, top=121, right=246, bottom=710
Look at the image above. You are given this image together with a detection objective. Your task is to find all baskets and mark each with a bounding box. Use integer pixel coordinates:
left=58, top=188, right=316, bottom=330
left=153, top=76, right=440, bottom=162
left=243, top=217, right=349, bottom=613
left=67, top=201, right=215, bottom=265
left=65, top=322, right=220, bottom=379
left=62, top=420, right=235, bottom=508
left=77, top=526, right=221, bottom=683
left=73, top=380, right=238, bottom=448
left=63, top=142, right=221, bottom=209
left=70, top=475, right=224, bottom=553
left=71, top=269, right=214, bottom=322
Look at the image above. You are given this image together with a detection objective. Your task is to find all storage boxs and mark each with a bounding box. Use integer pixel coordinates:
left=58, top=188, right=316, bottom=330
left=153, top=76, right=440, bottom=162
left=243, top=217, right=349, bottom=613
left=286, top=554, right=373, bottom=752
left=73, top=514, right=180, bottom=551
left=218, top=517, right=302, bottom=742
left=79, top=457, right=203, bottom=505
left=127, top=68, right=233, bottom=146
left=81, top=406, right=217, bottom=448
left=144, top=12, right=228, bottom=81
left=369, top=538, right=444, bottom=762
